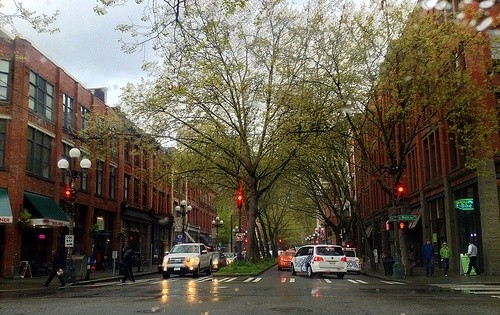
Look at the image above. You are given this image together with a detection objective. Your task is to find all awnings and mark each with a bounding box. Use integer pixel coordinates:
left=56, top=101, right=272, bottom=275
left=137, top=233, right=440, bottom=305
left=22, top=191, right=75, bottom=228
left=0, top=188, right=14, bottom=224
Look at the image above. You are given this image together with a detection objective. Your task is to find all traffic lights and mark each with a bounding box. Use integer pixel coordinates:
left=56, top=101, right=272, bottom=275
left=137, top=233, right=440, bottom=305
left=399, top=223, right=404, bottom=228
left=238, top=196, right=242, bottom=200
left=399, top=187, right=403, bottom=192
left=65, top=190, right=70, bottom=195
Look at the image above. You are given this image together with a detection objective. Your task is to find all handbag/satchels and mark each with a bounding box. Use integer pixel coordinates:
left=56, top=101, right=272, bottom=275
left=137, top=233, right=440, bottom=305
left=57, top=268, right=64, bottom=276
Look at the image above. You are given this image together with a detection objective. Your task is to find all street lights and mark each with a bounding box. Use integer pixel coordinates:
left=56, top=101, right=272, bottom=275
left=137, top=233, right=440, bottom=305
left=175, top=200, right=192, bottom=242
left=305, top=225, right=323, bottom=243
left=212, top=217, right=223, bottom=248
left=57, top=148, right=92, bottom=284
left=233, top=226, right=239, bottom=241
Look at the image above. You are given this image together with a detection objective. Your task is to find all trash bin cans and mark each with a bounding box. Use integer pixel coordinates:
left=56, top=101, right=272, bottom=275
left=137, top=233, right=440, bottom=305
left=383, top=261, right=395, bottom=276
left=71, top=255, right=87, bottom=281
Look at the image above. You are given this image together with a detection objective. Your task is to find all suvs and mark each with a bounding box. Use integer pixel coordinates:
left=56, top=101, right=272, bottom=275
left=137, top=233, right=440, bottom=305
left=162, top=243, right=212, bottom=278
left=290, top=244, right=348, bottom=278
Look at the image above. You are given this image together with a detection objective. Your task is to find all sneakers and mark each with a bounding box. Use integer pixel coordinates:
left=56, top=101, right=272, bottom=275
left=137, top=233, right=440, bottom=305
left=41, top=284, right=48, bottom=288
left=59, top=285, right=66, bottom=288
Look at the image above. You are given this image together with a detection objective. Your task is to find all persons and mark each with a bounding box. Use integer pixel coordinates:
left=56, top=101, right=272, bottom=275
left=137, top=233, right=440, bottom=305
left=119, top=245, right=137, bottom=283
left=407, top=243, right=417, bottom=274
left=374, top=243, right=381, bottom=268
left=464, top=240, right=482, bottom=277
left=421, top=239, right=436, bottom=277
left=43, top=244, right=67, bottom=287
left=439, top=242, right=453, bottom=277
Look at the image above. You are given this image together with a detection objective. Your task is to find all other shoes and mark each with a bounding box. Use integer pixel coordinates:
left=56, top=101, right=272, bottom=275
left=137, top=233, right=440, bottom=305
left=426, top=274, right=429, bottom=277
left=445, top=272, right=447, bottom=276
left=477, top=274, right=480, bottom=276
left=464, top=273, right=469, bottom=275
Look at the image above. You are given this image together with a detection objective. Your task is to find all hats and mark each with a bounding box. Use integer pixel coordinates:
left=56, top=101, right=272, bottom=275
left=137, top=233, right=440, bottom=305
left=443, top=242, right=447, bottom=245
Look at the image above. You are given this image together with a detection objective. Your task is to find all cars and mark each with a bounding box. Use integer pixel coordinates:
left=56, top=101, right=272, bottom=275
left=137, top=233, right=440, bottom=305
left=211, top=252, right=226, bottom=271
left=222, top=253, right=238, bottom=265
left=342, top=248, right=361, bottom=274
left=278, top=251, right=295, bottom=271
